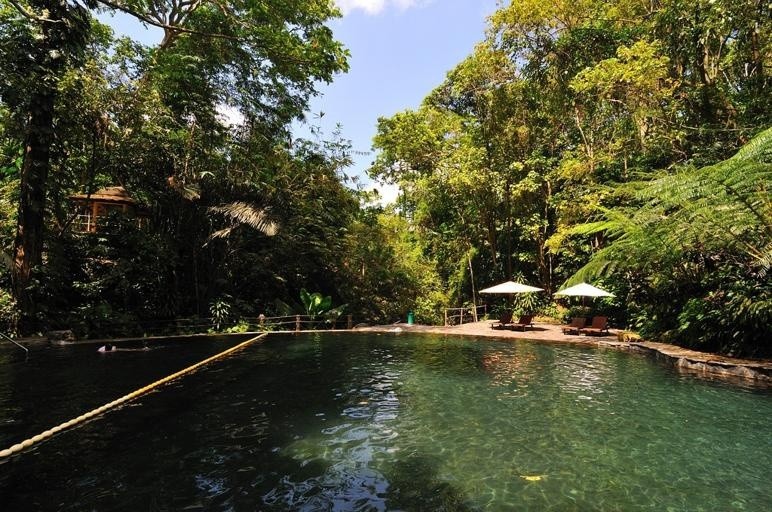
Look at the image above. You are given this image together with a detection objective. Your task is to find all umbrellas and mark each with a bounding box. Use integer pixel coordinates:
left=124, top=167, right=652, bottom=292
left=550, top=280, right=620, bottom=312
left=478, top=280, right=546, bottom=311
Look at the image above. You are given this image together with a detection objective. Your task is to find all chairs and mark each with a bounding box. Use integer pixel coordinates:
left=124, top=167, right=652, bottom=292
left=562, top=315, right=613, bottom=338
left=490, top=311, right=537, bottom=333
